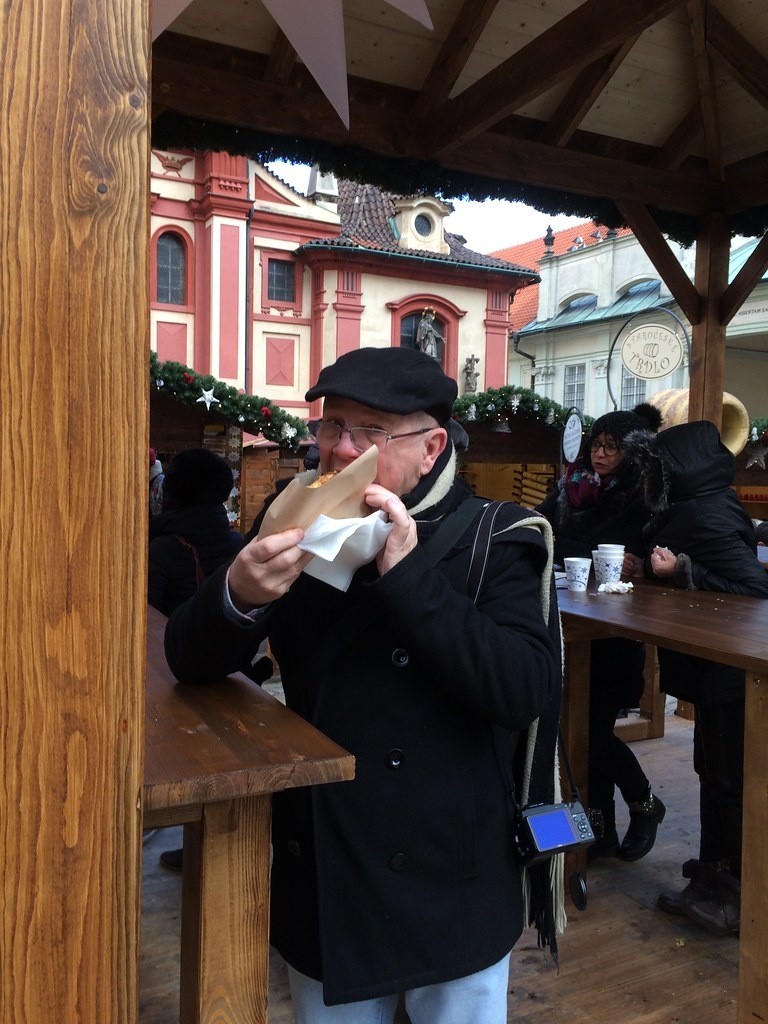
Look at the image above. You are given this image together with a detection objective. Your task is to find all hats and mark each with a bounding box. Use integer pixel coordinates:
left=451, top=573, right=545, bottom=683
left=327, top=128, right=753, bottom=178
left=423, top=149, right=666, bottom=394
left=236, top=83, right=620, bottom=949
left=304, top=346, right=459, bottom=425
left=589, top=402, right=664, bottom=446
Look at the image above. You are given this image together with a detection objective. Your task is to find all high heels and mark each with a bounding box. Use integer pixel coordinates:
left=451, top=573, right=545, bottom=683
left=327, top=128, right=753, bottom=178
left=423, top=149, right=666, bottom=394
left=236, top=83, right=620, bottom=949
left=587, top=800, right=620, bottom=854
left=619, top=785, right=667, bottom=861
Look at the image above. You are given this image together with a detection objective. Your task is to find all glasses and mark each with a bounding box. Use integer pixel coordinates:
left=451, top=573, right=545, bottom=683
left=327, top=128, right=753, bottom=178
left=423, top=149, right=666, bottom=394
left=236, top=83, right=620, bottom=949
left=588, top=439, right=626, bottom=456
left=307, top=416, right=438, bottom=452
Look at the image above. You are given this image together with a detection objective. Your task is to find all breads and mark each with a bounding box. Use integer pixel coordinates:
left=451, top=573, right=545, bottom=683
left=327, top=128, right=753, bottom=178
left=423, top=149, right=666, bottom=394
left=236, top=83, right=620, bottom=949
left=308, top=471, right=337, bottom=488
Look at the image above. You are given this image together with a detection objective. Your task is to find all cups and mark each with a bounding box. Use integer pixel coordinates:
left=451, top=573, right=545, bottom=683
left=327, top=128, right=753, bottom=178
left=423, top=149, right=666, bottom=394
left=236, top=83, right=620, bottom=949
left=563, top=557, right=592, bottom=592
left=592, top=544, right=626, bottom=587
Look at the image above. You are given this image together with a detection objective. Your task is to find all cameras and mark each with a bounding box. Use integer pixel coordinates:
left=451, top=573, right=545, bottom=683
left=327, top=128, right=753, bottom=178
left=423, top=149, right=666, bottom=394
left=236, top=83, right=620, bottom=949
left=517, top=800, right=598, bottom=869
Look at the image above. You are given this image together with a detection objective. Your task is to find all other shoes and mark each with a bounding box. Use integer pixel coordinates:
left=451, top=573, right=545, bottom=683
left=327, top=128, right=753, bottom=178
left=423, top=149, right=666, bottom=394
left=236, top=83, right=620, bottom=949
left=686, top=898, right=740, bottom=933
left=658, top=882, right=709, bottom=916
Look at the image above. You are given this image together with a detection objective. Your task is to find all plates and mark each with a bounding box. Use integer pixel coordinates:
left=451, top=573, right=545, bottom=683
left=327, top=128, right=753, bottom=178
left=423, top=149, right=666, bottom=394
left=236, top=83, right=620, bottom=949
left=555, top=571, right=567, bottom=588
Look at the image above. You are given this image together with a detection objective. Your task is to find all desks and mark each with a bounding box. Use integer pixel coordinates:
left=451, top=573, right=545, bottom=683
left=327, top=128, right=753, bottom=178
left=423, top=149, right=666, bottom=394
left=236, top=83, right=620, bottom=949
left=555, top=583, right=768, bottom=1024
left=141, top=601, right=372, bottom=1024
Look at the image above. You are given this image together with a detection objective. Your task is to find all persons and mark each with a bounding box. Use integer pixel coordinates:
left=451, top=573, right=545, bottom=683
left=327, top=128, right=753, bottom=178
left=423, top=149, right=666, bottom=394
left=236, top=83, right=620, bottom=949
left=624, top=421, right=768, bottom=937
left=164, top=346, right=566, bottom=1024
left=416, top=314, right=447, bottom=358
left=754, top=522, right=768, bottom=562
left=149, top=448, right=165, bottom=520
left=147, top=447, right=273, bottom=688
left=531, top=403, right=667, bottom=862
